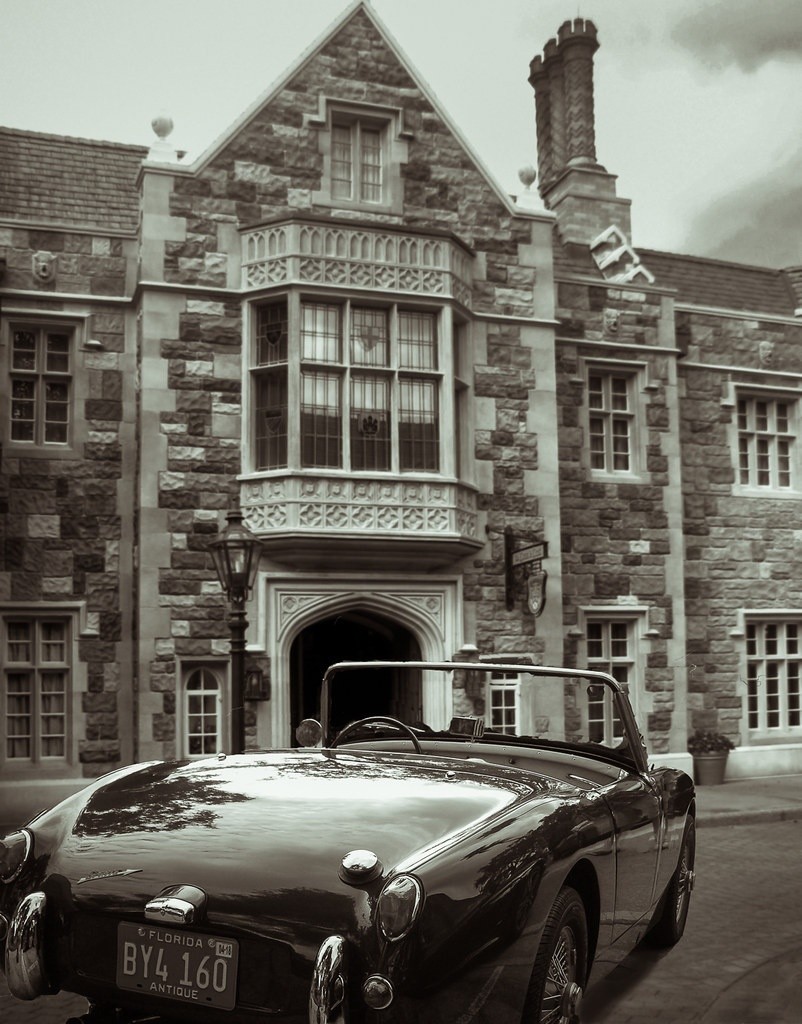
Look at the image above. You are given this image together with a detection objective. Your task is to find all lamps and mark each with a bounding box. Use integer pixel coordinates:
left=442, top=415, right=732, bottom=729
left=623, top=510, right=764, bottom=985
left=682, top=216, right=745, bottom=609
left=249, top=665, right=272, bottom=701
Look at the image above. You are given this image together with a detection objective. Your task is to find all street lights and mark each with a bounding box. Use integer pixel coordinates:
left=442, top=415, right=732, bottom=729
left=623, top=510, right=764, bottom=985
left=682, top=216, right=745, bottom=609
left=204, top=497, right=265, bottom=753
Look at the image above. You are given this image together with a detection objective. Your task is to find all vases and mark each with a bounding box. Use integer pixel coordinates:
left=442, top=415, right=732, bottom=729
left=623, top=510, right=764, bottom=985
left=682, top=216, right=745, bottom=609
left=693, top=753, right=727, bottom=785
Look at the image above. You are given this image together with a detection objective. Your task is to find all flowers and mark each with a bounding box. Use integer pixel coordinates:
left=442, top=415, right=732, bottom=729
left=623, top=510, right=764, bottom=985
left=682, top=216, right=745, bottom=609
left=689, top=728, right=734, bottom=752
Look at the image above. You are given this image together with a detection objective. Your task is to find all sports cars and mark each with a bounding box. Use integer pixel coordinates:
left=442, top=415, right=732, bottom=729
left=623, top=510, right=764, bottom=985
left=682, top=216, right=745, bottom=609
left=0, top=660, right=697, bottom=1024
